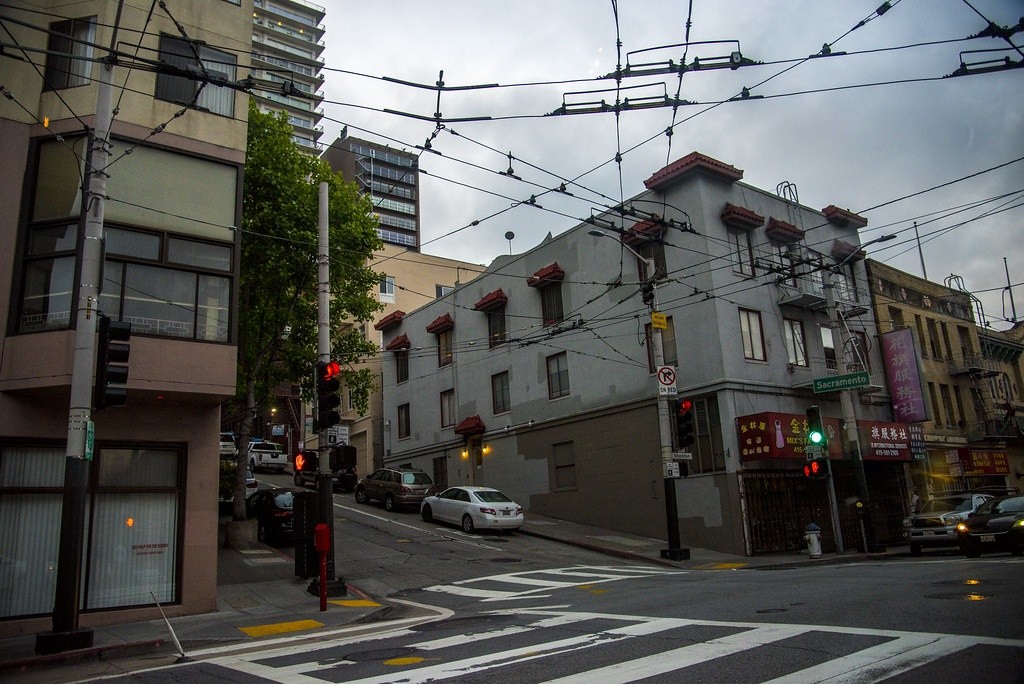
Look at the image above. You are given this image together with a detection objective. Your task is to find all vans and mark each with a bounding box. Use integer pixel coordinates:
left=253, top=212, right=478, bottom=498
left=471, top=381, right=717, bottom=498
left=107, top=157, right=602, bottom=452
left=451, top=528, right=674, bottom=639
left=220, top=432, right=237, bottom=460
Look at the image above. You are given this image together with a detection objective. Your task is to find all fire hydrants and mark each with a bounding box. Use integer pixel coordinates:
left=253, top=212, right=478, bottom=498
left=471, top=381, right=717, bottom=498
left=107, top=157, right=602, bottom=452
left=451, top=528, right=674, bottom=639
left=803, top=522, right=823, bottom=559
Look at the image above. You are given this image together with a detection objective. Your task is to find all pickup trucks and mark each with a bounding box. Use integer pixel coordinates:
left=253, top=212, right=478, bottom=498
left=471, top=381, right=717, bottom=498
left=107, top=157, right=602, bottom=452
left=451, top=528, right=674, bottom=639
left=232, top=441, right=288, bottom=474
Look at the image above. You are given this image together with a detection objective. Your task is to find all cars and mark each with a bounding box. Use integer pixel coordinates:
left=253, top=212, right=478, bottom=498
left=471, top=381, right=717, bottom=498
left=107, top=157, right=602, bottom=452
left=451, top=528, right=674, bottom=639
left=420, top=485, right=524, bottom=533
left=294, top=457, right=358, bottom=494
left=245, top=487, right=299, bottom=548
left=957, top=495, right=1024, bottom=557
left=219, top=461, right=260, bottom=512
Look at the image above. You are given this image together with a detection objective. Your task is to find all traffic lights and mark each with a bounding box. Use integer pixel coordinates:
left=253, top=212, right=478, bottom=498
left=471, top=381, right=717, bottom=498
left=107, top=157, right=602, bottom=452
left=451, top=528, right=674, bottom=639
left=92, top=316, right=131, bottom=409
left=803, top=458, right=823, bottom=478
left=293, top=452, right=309, bottom=472
left=314, top=360, right=341, bottom=429
left=342, top=446, right=357, bottom=466
left=804, top=408, right=821, bottom=444
left=855, top=500, right=864, bottom=516
left=675, top=398, right=695, bottom=447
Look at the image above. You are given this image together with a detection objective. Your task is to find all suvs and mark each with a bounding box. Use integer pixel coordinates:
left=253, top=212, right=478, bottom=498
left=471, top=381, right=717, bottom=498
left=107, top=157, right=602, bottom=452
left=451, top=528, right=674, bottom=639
left=908, top=493, right=995, bottom=557
left=355, top=466, right=437, bottom=512
